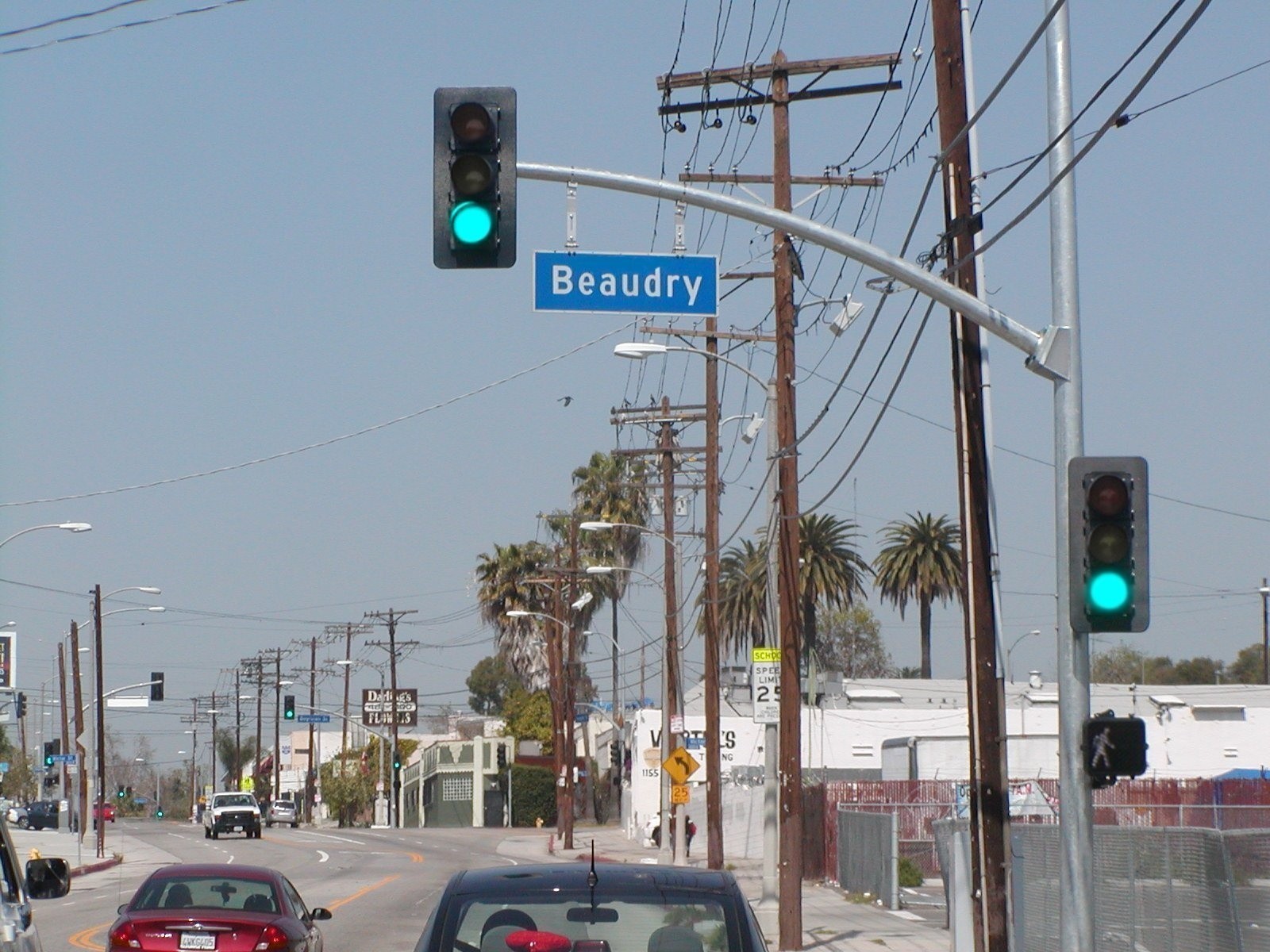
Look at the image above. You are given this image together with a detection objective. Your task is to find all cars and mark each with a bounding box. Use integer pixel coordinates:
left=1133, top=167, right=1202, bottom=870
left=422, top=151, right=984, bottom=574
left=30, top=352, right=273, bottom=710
left=413, top=861, right=765, bottom=952
left=7, top=801, right=98, bottom=833
left=108, top=863, right=332, bottom=952
left=92, top=802, right=118, bottom=822
left=0, top=814, right=72, bottom=952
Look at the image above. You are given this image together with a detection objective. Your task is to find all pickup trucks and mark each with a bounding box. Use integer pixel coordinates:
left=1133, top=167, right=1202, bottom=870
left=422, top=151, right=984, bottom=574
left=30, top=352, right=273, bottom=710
left=201, top=792, right=262, bottom=840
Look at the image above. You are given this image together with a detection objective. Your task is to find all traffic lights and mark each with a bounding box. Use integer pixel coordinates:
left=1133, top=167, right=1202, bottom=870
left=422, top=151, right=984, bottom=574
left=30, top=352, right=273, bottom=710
left=44, top=742, right=54, bottom=767
left=284, top=695, right=295, bottom=719
left=1082, top=713, right=1149, bottom=784
left=393, top=751, right=401, bottom=776
left=1062, top=455, right=1148, bottom=633
left=434, top=85, right=519, bottom=269
left=18, top=691, right=27, bottom=718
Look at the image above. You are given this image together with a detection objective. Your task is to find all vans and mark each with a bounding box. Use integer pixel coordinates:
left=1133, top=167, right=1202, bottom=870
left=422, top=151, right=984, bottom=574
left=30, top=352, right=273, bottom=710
left=265, top=799, right=297, bottom=829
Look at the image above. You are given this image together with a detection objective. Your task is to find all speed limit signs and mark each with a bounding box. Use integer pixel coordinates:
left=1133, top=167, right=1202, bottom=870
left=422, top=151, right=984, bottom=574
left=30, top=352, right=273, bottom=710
left=752, top=650, right=784, bottom=726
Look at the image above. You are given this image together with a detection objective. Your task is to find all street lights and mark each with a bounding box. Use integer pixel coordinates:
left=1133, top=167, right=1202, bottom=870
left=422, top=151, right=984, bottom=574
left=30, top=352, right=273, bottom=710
left=278, top=681, right=322, bottom=826
left=239, top=694, right=277, bottom=801
left=38, top=584, right=167, bottom=858
left=178, top=750, right=197, bottom=817
left=335, top=659, right=388, bottom=827
left=503, top=519, right=689, bottom=866
left=134, top=758, right=161, bottom=812
left=610, top=341, right=803, bottom=952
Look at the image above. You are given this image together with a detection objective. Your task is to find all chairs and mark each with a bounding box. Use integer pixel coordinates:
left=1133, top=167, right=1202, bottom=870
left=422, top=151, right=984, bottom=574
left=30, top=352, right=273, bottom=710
left=648, top=927, right=703, bottom=952
left=480, top=910, right=537, bottom=952
left=243, top=894, right=272, bottom=911
left=165, top=884, right=192, bottom=907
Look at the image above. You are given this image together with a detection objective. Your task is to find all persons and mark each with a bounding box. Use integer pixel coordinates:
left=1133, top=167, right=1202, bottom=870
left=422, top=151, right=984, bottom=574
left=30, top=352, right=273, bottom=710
left=168, top=884, right=193, bottom=905
left=480, top=909, right=538, bottom=948
